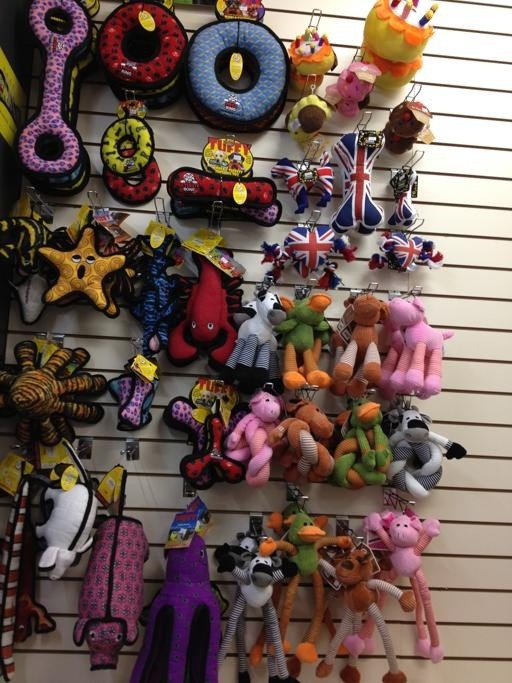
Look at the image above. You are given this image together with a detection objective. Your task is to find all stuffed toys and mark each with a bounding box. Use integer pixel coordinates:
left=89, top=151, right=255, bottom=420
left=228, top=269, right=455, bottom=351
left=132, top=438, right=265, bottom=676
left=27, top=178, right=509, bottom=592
left=168, top=250, right=469, bottom=496
left=1, top=476, right=469, bottom=681
left=1, top=1, right=468, bottom=681
